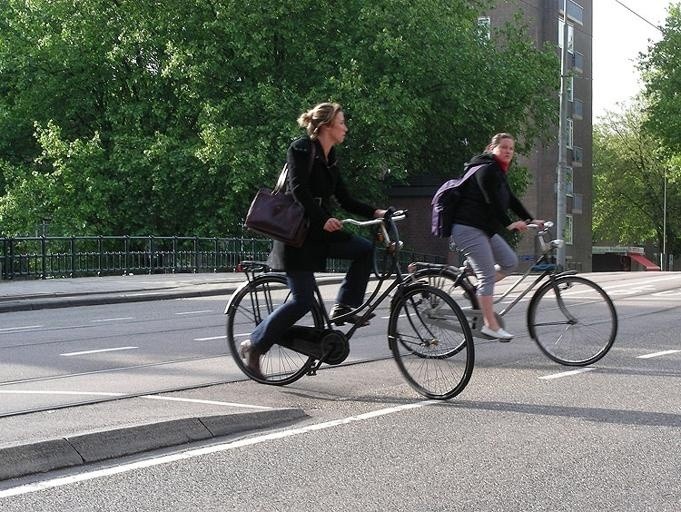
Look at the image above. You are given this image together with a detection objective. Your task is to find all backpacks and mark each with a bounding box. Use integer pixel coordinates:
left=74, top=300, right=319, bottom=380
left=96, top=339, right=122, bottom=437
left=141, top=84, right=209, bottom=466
left=430, top=163, right=488, bottom=239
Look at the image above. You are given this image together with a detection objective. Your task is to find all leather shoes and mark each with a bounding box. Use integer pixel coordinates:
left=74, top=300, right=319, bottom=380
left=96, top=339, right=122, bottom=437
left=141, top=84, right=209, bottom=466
left=480, top=325, right=514, bottom=340
left=238, top=339, right=266, bottom=379
left=328, top=304, right=377, bottom=327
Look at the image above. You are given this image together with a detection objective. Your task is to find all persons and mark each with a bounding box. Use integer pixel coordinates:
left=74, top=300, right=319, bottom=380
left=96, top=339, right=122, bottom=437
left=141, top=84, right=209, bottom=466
left=450, top=133, right=547, bottom=340
left=237, top=102, right=405, bottom=381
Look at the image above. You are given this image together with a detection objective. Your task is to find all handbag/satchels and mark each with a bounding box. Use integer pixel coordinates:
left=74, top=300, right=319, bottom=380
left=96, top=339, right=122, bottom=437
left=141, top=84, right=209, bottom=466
left=243, top=187, right=314, bottom=250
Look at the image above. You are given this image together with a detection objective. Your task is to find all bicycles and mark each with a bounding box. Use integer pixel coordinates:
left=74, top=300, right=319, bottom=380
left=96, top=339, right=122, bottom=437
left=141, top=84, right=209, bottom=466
left=390, top=218, right=618, bottom=367
left=224, top=207, right=475, bottom=402
